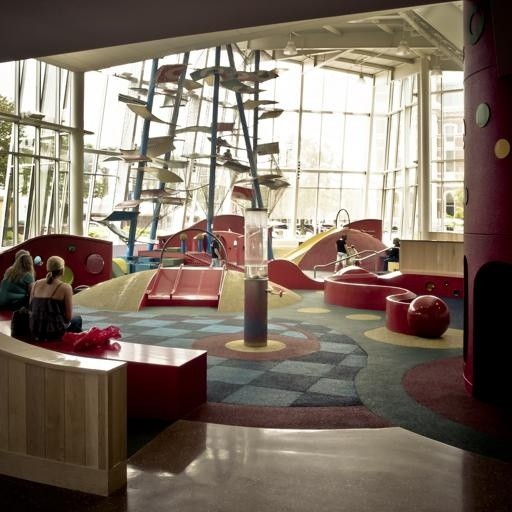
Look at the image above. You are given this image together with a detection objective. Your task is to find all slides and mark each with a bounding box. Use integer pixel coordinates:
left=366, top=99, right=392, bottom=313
left=145, top=264, right=226, bottom=306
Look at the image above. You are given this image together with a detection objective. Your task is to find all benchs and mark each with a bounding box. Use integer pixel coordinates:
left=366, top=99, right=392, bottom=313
left=0, top=308, right=209, bottom=413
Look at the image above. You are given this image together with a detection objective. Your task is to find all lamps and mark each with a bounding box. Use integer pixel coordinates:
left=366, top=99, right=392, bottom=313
left=282, top=32, right=298, bottom=56
left=395, top=20, right=411, bottom=56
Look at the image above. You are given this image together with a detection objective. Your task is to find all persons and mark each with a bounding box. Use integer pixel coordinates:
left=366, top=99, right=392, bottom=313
left=210, top=234, right=221, bottom=266
left=30, top=256, right=82, bottom=344
left=1, top=254, right=34, bottom=310
left=334, top=233, right=349, bottom=272
left=383, top=238, right=400, bottom=270
left=6, top=249, right=37, bottom=281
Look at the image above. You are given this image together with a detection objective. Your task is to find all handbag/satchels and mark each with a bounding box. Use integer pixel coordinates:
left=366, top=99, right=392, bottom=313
left=11, top=307, right=33, bottom=336
